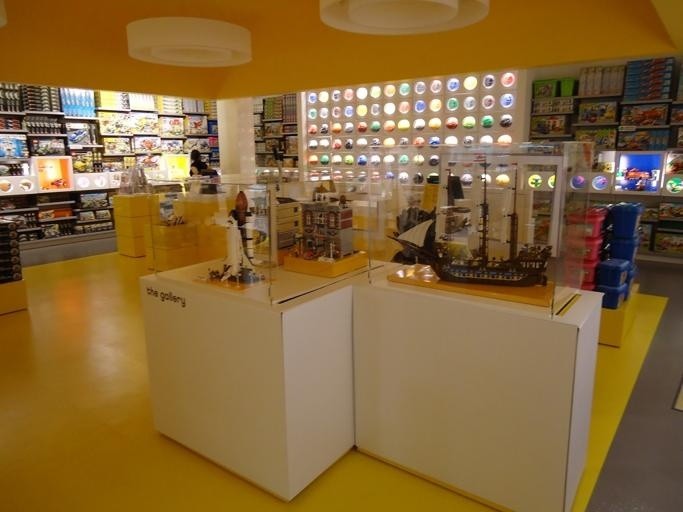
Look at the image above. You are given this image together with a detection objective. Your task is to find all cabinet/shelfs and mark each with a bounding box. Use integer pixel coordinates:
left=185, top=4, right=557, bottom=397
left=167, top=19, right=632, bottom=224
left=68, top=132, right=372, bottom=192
left=0, top=53, right=683, bottom=262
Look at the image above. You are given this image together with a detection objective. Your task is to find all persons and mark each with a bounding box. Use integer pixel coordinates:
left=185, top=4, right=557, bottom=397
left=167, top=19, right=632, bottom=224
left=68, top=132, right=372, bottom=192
left=188, top=148, right=208, bottom=176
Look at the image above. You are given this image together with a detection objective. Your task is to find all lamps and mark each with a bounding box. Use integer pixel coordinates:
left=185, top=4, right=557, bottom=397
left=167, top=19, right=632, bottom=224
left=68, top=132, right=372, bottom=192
left=125, top=17, right=254, bottom=67
left=317, top=1, right=490, bottom=36
